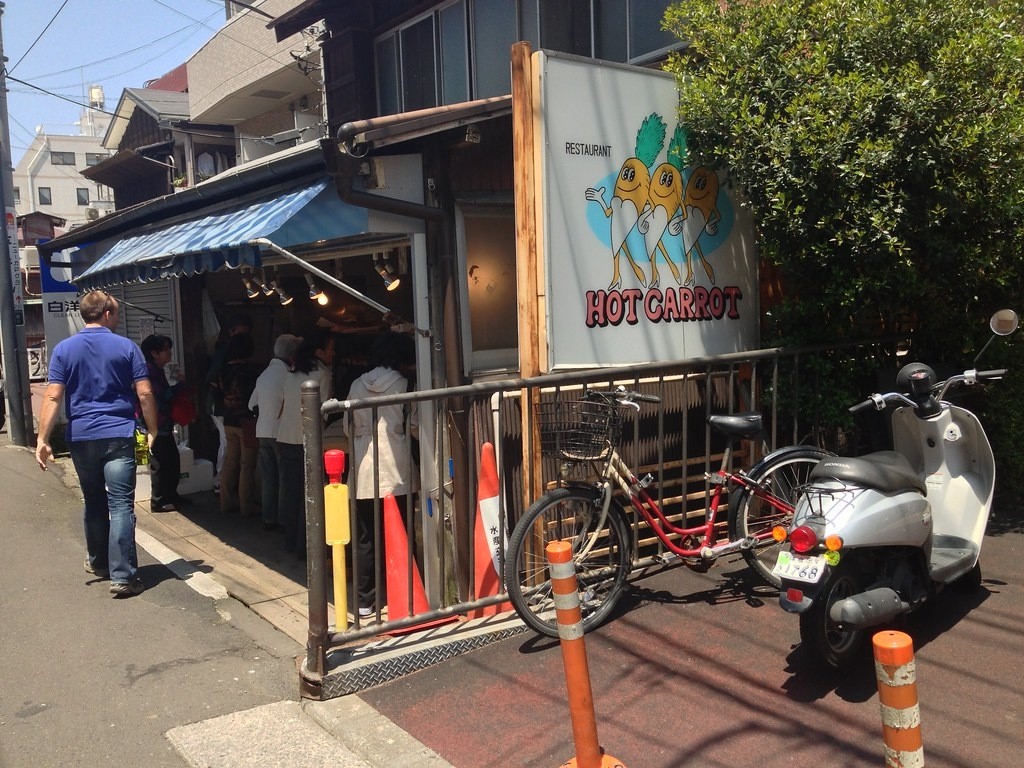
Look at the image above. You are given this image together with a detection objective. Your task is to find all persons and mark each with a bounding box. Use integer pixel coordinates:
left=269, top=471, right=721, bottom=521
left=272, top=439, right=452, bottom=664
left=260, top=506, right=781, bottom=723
left=36, top=292, right=159, bottom=595
left=141, top=314, right=419, bottom=616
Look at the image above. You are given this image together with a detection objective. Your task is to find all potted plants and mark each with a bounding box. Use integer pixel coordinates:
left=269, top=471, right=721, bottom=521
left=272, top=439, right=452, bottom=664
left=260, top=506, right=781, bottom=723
left=170, top=172, right=188, bottom=187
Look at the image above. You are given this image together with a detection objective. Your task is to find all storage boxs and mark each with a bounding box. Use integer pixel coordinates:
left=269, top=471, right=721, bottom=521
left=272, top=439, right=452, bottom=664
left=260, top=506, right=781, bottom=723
left=178, top=446, right=195, bottom=468
left=177, top=458, right=214, bottom=496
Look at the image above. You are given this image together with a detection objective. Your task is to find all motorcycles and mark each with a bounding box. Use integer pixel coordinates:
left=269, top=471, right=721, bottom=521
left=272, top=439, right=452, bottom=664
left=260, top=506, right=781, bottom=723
left=770, top=310, right=1018, bottom=670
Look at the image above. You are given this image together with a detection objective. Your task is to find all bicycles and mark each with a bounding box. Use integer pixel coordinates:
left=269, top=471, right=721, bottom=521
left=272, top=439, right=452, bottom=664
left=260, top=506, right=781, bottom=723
left=503, top=384, right=848, bottom=642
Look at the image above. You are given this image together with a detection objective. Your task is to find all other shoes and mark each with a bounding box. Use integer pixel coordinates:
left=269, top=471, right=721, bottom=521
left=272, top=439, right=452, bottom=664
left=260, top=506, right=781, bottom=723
left=264, top=521, right=287, bottom=532
left=215, top=483, right=221, bottom=494
left=358, top=600, right=376, bottom=616
left=150, top=503, right=186, bottom=513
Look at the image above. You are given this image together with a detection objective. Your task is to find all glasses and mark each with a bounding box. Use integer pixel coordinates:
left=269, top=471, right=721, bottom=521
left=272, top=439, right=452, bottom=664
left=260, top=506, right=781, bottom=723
left=82, top=289, right=113, bottom=310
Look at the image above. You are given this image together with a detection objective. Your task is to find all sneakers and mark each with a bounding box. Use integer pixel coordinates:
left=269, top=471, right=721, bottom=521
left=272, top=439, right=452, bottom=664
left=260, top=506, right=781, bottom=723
left=109, top=577, right=146, bottom=595
left=83, top=559, right=110, bottom=578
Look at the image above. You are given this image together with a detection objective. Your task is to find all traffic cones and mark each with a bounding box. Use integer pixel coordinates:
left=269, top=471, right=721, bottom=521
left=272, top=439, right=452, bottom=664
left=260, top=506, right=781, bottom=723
left=464, top=441, right=515, bottom=623
left=377, top=493, right=460, bottom=639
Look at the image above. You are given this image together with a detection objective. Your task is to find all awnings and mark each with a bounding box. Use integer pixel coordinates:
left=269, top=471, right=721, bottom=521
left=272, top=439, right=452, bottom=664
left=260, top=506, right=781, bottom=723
left=72, top=173, right=370, bottom=296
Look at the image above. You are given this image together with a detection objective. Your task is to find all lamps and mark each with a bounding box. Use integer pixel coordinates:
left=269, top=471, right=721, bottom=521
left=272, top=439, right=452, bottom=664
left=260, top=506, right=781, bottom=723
left=242, top=276, right=259, bottom=299
left=375, top=264, right=400, bottom=291
left=275, top=288, right=294, bottom=305
left=252, top=275, right=273, bottom=296
left=304, top=272, right=324, bottom=300
left=265, top=270, right=283, bottom=293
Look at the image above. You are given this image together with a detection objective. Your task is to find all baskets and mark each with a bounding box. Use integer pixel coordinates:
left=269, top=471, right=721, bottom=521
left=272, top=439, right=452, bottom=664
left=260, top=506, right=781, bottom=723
left=532, top=399, right=630, bottom=462
